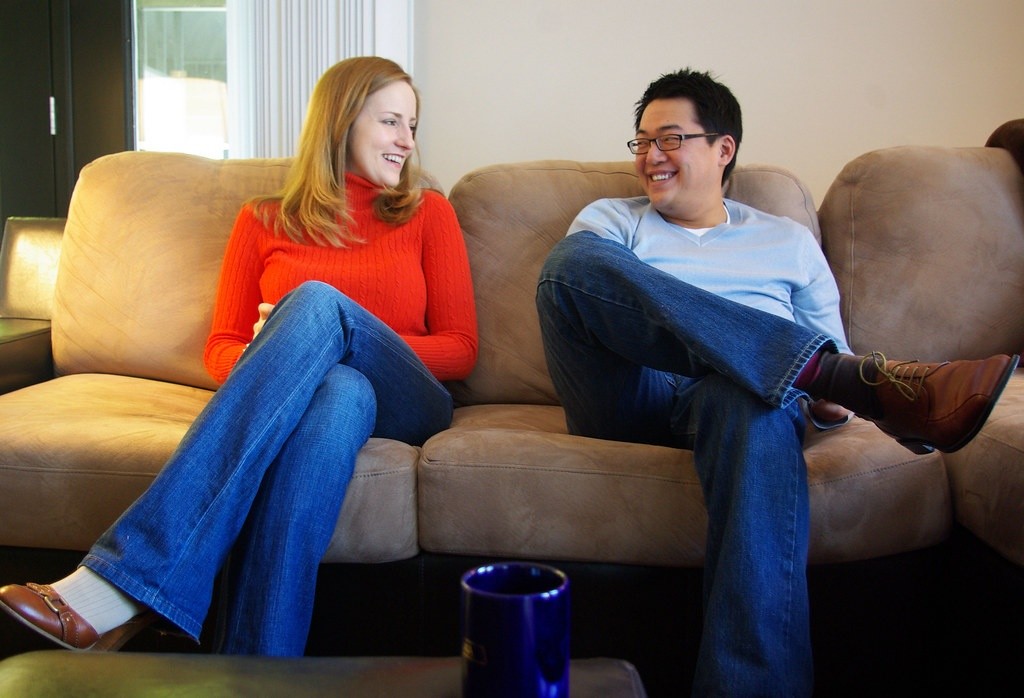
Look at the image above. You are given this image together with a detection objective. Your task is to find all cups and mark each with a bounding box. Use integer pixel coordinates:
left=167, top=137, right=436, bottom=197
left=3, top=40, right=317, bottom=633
left=460, top=561, right=571, bottom=698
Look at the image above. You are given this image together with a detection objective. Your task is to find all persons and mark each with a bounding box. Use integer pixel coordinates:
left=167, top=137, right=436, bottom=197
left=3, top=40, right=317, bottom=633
left=534, top=68, right=1019, bottom=698
left=0, top=56, right=479, bottom=659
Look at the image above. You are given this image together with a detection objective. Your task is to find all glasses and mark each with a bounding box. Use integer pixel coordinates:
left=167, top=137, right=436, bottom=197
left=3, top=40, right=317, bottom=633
left=624, top=132, right=720, bottom=156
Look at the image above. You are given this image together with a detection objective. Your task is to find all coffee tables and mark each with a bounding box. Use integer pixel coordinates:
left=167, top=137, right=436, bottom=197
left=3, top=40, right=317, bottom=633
left=0, top=648, right=647, bottom=698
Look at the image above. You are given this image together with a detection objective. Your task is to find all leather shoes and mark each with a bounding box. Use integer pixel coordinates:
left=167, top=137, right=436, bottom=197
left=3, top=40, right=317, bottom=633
left=874, top=351, right=1022, bottom=456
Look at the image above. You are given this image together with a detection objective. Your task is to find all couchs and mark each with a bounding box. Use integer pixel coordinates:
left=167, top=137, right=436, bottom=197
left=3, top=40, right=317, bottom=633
left=818, top=144, right=1024, bottom=568
left=0, top=151, right=445, bottom=566
left=418, top=160, right=953, bottom=567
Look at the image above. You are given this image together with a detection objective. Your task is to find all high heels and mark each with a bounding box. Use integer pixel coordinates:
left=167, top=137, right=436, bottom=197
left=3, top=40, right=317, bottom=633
left=0, top=578, right=136, bottom=650
